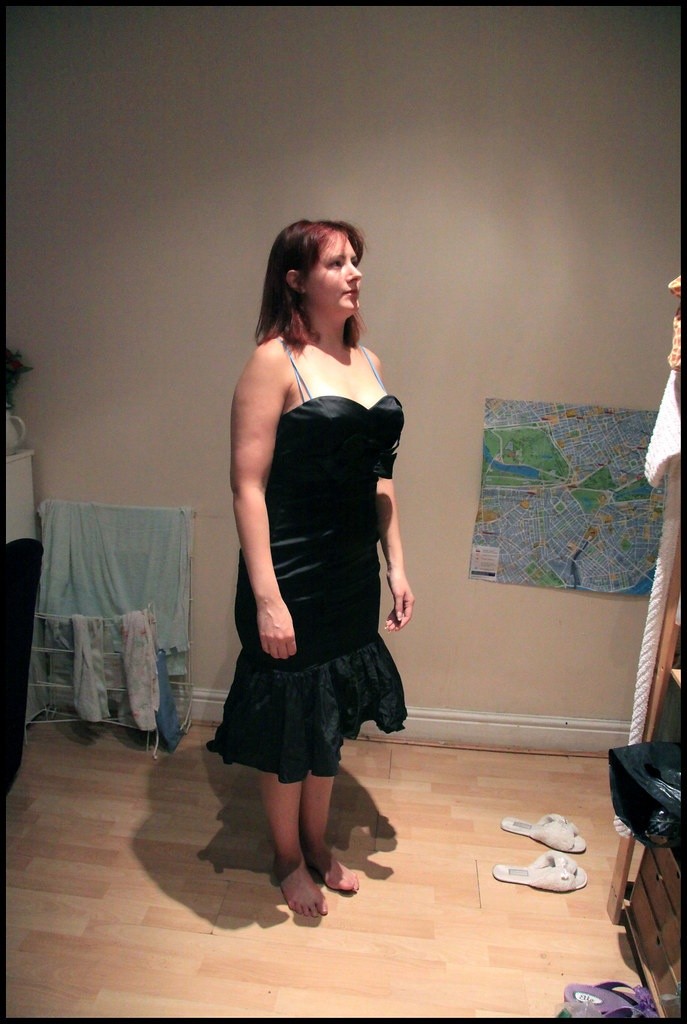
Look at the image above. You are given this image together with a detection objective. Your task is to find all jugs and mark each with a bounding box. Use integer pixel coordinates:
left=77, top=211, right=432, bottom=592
left=6, top=402, right=25, bottom=455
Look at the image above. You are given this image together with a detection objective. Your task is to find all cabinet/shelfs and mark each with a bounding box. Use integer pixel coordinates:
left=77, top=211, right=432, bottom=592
left=624, top=834, right=681, bottom=1018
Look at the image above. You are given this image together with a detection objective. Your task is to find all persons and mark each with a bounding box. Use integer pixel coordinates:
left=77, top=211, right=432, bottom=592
left=204, top=218, right=415, bottom=917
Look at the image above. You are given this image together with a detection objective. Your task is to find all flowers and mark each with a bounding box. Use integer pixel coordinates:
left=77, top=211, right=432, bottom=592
left=4, top=348, right=35, bottom=409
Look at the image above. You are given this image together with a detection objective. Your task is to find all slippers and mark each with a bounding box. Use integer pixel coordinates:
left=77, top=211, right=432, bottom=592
left=602, top=1004, right=661, bottom=1018
left=501, top=814, right=587, bottom=853
left=564, top=981, right=657, bottom=1014
left=492, top=850, right=587, bottom=892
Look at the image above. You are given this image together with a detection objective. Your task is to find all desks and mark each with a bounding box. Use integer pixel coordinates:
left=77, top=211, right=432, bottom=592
left=4, top=449, right=52, bottom=726
